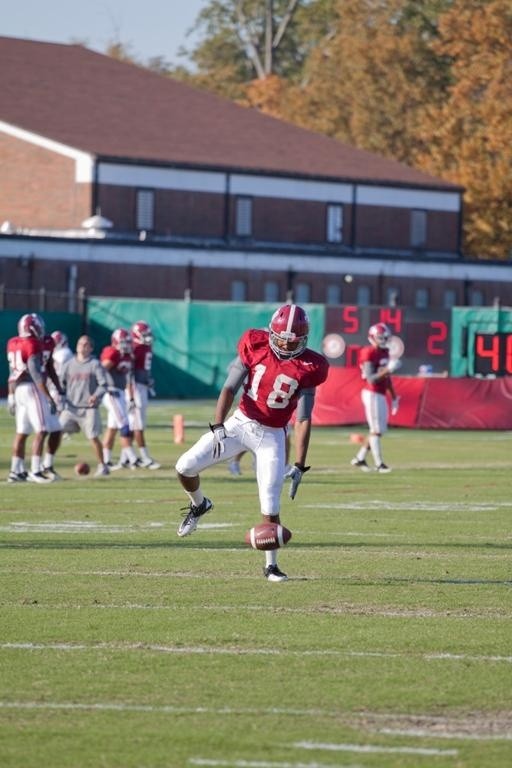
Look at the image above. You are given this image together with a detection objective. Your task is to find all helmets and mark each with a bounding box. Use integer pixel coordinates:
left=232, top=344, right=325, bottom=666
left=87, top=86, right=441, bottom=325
left=131, top=320, right=153, bottom=345
left=18, top=313, right=46, bottom=339
left=267, top=305, right=309, bottom=360
left=367, top=323, right=390, bottom=348
left=111, top=329, right=132, bottom=353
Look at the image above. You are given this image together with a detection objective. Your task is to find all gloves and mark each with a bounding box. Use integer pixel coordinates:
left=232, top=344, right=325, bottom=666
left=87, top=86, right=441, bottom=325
left=46, top=392, right=67, bottom=415
left=283, top=462, right=311, bottom=500
left=388, top=358, right=404, bottom=374
left=7, top=393, right=17, bottom=415
left=209, top=422, right=236, bottom=459
left=390, top=397, right=400, bottom=417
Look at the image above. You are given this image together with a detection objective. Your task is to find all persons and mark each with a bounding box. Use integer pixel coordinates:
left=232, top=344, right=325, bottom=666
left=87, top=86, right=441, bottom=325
left=228, top=420, right=292, bottom=478
left=176, top=303, right=329, bottom=583
left=7, top=312, right=161, bottom=485
left=350, top=323, right=403, bottom=475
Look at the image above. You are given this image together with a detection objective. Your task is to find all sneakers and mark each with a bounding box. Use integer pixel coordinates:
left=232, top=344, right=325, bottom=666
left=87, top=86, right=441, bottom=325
left=177, top=497, right=213, bottom=537
left=350, top=457, right=372, bottom=472
left=226, top=460, right=243, bottom=476
left=262, top=564, right=289, bottom=581
left=7, top=462, right=61, bottom=483
left=374, top=464, right=393, bottom=473
left=95, top=457, right=161, bottom=476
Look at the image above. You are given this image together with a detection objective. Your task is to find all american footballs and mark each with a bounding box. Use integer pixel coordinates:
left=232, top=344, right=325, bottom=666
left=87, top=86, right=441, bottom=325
left=76, top=462, right=90, bottom=476
left=245, top=521, right=292, bottom=550
